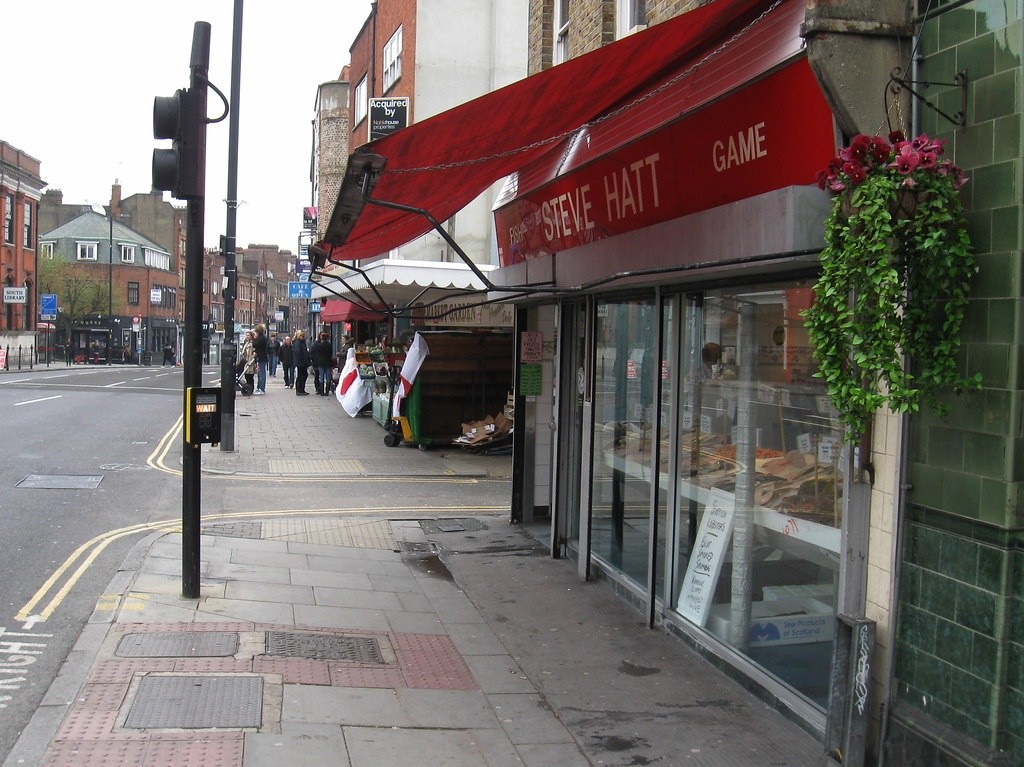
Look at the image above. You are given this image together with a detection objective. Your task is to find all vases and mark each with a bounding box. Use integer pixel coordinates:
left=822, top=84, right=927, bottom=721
left=839, top=185, right=930, bottom=231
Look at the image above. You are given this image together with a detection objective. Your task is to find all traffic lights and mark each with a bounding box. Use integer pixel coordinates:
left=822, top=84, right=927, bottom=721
left=150, top=88, right=206, bottom=201
left=132, top=316, right=141, bottom=324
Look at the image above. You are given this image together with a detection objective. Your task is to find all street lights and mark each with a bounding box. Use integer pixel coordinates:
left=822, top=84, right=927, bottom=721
left=249, top=274, right=263, bottom=330
left=107, top=214, right=133, bottom=366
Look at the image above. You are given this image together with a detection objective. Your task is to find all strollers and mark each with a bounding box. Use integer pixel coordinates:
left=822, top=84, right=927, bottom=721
left=217, top=357, right=257, bottom=397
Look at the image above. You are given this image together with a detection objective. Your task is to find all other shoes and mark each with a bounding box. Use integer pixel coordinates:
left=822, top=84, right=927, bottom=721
left=296, top=392, right=309, bottom=396
left=256, top=388, right=265, bottom=393
left=285, top=384, right=289, bottom=388
left=289, top=384, right=293, bottom=389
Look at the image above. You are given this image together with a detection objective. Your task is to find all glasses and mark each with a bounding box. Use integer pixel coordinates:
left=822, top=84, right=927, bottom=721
left=300, top=335, right=305, bottom=338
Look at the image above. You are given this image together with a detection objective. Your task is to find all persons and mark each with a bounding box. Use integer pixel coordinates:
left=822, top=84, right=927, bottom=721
left=314, top=333, right=333, bottom=396
left=292, top=330, right=311, bottom=395
left=266, top=334, right=280, bottom=377
left=241, top=331, right=257, bottom=390
left=684, top=342, right=723, bottom=431
left=91, top=340, right=100, bottom=352
left=336, top=335, right=354, bottom=373
left=64, top=339, right=71, bottom=366
left=310, top=332, right=322, bottom=394
left=252, top=325, right=268, bottom=395
left=279, top=336, right=295, bottom=389
left=161, top=342, right=176, bottom=368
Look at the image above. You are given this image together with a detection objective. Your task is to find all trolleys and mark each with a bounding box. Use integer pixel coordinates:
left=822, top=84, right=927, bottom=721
left=382, top=366, right=430, bottom=451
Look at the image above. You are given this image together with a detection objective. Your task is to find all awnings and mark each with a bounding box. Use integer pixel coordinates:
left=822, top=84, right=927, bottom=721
left=323, top=0, right=766, bottom=261
left=310, top=258, right=496, bottom=306
left=323, top=300, right=385, bottom=323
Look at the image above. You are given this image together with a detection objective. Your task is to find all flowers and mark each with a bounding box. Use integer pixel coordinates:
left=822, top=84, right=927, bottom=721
left=801, top=128, right=981, bottom=447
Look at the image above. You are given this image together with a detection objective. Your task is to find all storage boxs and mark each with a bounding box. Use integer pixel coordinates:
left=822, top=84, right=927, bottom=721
left=706, top=583, right=835, bottom=649
left=354, top=351, right=391, bottom=381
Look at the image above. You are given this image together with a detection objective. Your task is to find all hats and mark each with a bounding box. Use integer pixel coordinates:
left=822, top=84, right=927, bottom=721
left=321, top=333, right=329, bottom=340
left=271, top=335, right=275, bottom=337
